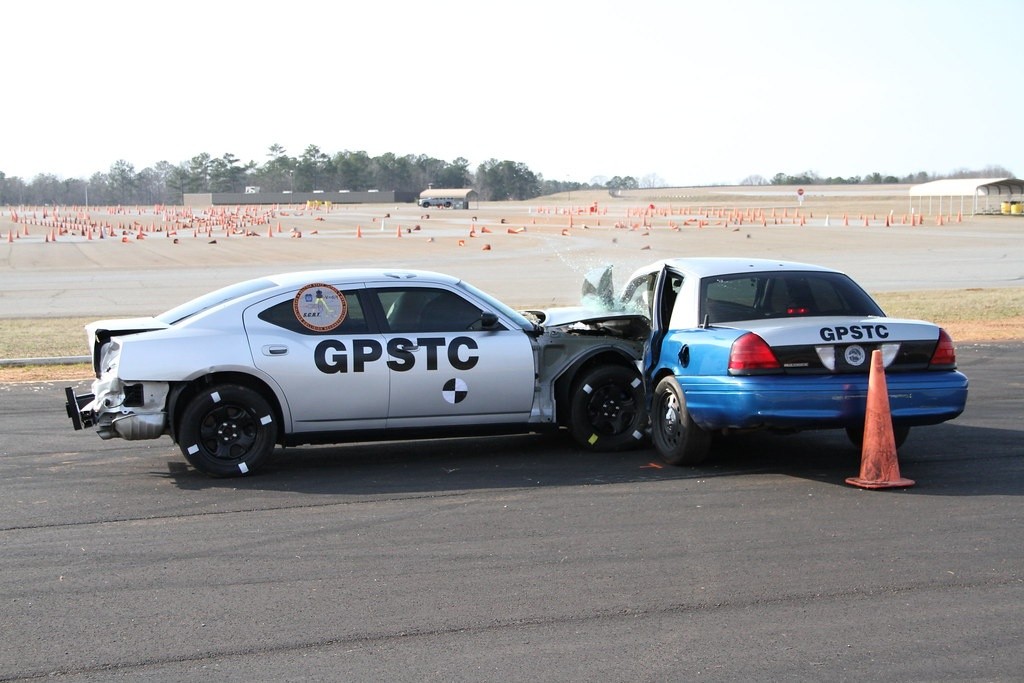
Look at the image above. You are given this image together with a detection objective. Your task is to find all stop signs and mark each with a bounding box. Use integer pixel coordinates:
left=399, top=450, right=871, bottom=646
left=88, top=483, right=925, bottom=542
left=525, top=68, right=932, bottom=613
left=798, top=189, right=804, bottom=195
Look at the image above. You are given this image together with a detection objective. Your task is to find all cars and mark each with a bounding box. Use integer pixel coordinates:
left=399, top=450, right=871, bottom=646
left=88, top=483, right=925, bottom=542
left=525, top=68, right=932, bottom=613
left=582, top=255, right=967, bottom=468
left=59, top=267, right=657, bottom=476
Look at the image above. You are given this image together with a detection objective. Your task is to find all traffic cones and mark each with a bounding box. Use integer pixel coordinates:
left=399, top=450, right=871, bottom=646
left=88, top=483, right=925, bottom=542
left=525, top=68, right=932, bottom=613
left=846, top=348, right=917, bottom=490
left=0, top=191, right=967, bottom=257
